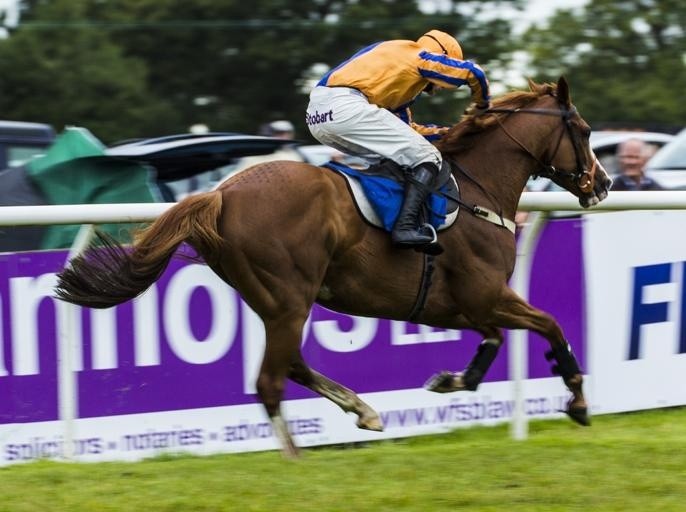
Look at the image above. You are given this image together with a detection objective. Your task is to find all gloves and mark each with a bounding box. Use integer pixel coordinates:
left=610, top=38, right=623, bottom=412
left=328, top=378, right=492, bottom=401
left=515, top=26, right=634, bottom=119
left=465, top=101, right=493, bottom=116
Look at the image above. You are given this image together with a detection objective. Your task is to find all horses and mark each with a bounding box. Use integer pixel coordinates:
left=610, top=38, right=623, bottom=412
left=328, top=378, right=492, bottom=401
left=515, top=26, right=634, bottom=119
left=52, top=74, right=614, bottom=462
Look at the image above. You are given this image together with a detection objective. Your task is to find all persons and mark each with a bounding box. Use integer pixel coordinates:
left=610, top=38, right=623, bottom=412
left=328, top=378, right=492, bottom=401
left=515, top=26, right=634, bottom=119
left=613, top=135, right=663, bottom=190
left=304, top=27, right=494, bottom=255
left=240, top=119, right=306, bottom=168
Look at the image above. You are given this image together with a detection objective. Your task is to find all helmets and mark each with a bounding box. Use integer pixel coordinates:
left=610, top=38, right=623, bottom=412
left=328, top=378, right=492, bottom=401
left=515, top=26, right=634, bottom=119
left=417, top=30, right=464, bottom=61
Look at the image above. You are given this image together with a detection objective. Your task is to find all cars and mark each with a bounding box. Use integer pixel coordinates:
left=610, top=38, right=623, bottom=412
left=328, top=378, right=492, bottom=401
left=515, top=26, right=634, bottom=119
left=524, top=128, right=686, bottom=198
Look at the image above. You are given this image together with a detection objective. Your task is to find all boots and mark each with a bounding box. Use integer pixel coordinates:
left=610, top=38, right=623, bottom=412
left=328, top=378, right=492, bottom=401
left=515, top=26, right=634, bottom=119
left=390, top=163, right=434, bottom=246
